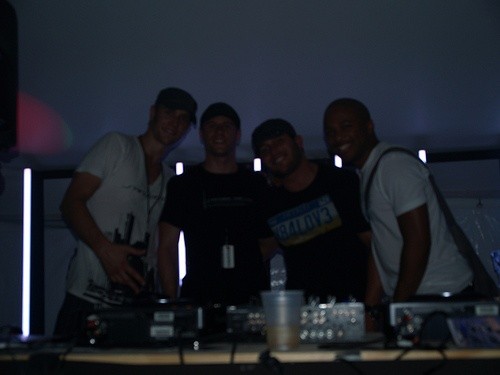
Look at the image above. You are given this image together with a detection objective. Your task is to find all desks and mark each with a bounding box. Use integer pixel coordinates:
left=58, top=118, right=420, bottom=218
left=0, top=347, right=500, bottom=365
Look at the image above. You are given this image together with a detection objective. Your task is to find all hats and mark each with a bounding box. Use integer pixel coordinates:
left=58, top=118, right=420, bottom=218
left=200, top=102, right=241, bottom=130
left=155, top=87, right=198, bottom=129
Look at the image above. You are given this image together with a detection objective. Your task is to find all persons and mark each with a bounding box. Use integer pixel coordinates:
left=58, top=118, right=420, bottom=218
left=320, top=96, right=500, bottom=332
left=50, top=86, right=200, bottom=339
left=251, top=116, right=372, bottom=305
left=157, top=101, right=277, bottom=313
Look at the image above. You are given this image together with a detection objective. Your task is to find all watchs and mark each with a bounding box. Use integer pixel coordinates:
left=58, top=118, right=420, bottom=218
left=363, top=304, right=381, bottom=319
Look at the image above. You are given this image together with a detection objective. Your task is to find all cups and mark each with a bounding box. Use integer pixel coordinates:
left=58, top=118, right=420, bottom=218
left=260, top=290, right=303, bottom=352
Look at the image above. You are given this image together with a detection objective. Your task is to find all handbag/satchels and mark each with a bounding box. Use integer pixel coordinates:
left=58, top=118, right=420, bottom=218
left=472, top=268, right=500, bottom=301
